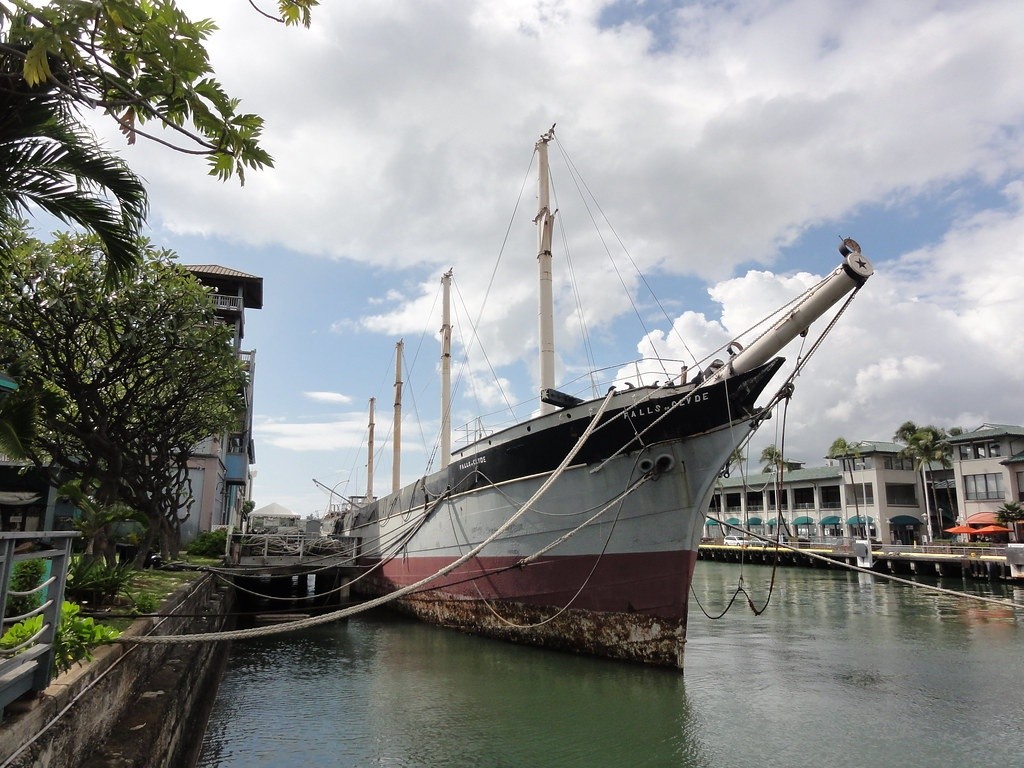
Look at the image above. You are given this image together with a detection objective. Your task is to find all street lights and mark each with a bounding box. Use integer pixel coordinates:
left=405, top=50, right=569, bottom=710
left=856, top=463, right=871, bottom=541
left=915, top=457, right=943, bottom=539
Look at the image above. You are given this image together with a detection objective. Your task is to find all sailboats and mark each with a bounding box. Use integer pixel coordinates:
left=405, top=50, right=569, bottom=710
left=324, top=127, right=875, bottom=674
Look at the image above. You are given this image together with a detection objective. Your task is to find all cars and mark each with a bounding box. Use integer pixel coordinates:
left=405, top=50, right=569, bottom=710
left=701, top=537, right=716, bottom=544
left=724, top=536, right=748, bottom=546
left=747, top=538, right=767, bottom=548
